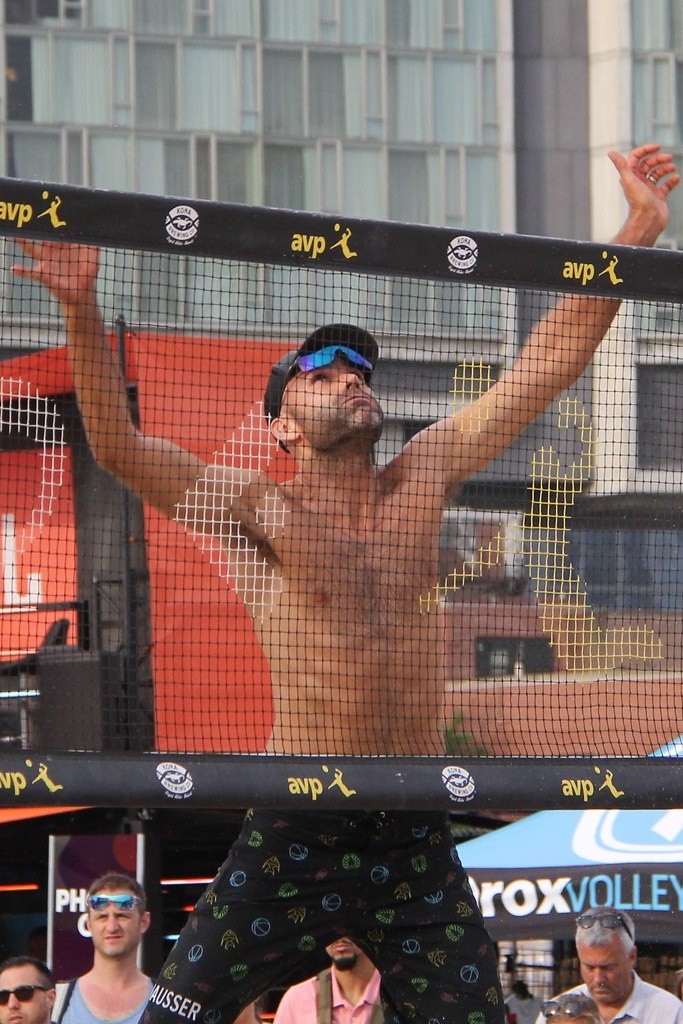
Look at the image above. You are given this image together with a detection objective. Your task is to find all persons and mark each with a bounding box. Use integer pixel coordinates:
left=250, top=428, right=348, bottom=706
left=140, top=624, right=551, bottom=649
left=11, top=144, right=682, bottom=1024
left=540, top=993, right=601, bottom=1024
left=0, top=953, right=56, bottom=1024
left=535, top=906, right=683, bottom=1024
left=273, top=936, right=384, bottom=1024
left=48, top=872, right=157, bottom=1024
left=505, top=981, right=540, bottom=1024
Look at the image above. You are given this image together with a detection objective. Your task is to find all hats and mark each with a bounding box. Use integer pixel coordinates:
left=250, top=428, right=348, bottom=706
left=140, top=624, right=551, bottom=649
left=263, top=323, right=379, bottom=454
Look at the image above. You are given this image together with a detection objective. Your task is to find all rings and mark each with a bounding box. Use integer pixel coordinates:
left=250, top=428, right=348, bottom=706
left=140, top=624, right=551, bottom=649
left=647, top=174, right=657, bottom=184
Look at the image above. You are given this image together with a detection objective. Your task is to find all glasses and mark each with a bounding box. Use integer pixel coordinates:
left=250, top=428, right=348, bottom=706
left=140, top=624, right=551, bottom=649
left=87, top=894, right=145, bottom=910
left=0, top=984, right=49, bottom=1005
left=277, top=342, right=373, bottom=419
left=574, top=915, right=633, bottom=940
left=541, top=1000, right=591, bottom=1018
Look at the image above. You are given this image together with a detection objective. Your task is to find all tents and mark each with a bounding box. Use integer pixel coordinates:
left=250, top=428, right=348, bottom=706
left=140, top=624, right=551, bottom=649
left=454, top=735, right=682, bottom=951
